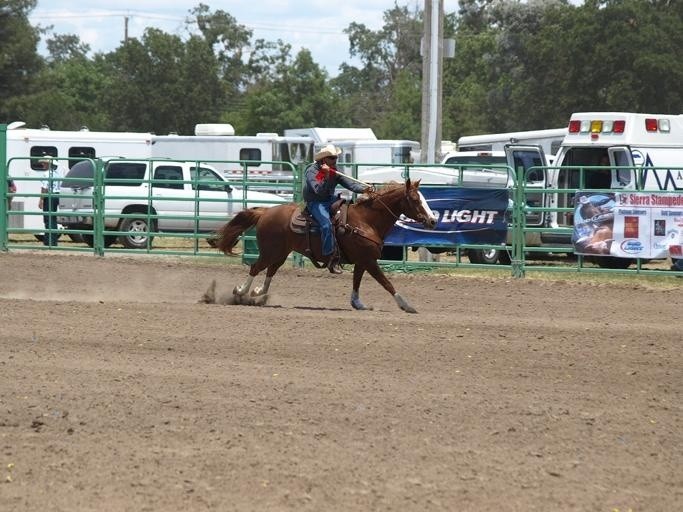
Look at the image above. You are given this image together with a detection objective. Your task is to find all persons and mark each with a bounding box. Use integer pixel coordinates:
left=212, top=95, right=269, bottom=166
left=8, top=180, right=16, bottom=211
left=577, top=195, right=616, bottom=220
left=302, top=144, right=368, bottom=275
left=39, top=155, right=60, bottom=246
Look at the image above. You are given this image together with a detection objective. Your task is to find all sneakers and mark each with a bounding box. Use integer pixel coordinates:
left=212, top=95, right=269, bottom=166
left=330, top=267, right=342, bottom=274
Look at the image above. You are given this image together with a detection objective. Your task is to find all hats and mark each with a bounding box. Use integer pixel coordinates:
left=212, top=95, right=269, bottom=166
left=38, top=155, right=57, bottom=167
left=315, top=144, right=342, bottom=161
left=576, top=196, right=590, bottom=204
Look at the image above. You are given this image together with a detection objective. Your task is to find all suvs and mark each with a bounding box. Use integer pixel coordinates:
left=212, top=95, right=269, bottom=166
left=56, top=158, right=290, bottom=250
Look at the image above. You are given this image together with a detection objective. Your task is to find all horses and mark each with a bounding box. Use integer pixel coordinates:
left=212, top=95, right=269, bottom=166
left=216, top=178, right=439, bottom=315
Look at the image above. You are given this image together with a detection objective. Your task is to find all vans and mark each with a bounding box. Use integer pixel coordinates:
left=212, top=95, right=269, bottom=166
left=504, top=108, right=681, bottom=271
left=441, top=150, right=556, bottom=265
